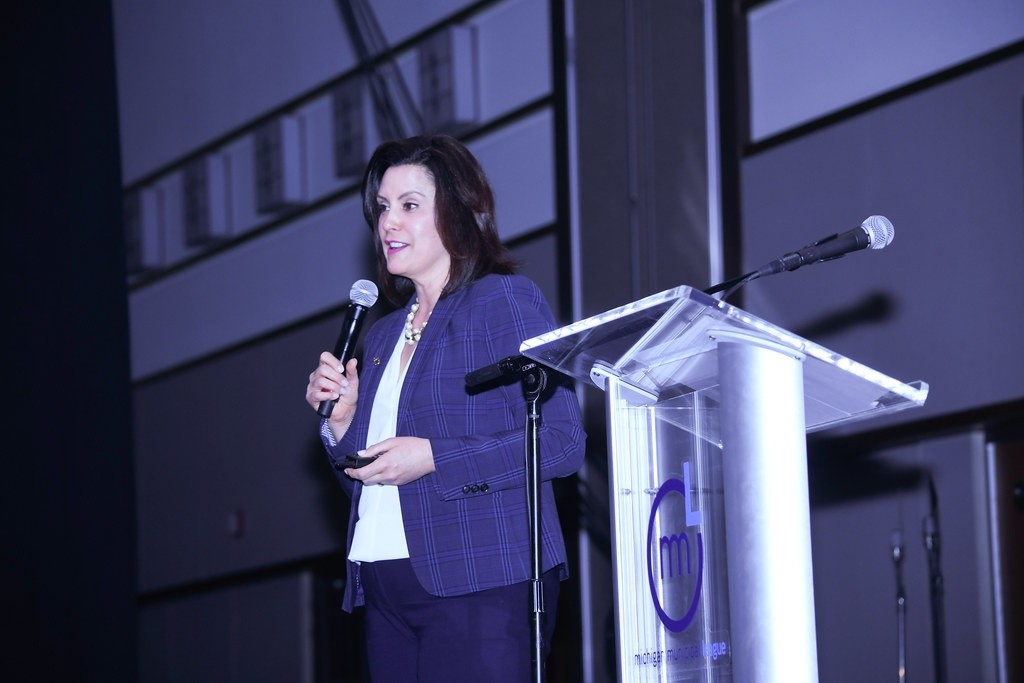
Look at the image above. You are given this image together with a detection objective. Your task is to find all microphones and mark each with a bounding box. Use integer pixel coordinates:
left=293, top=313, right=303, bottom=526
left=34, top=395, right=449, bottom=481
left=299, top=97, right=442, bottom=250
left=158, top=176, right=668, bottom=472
left=316, top=279, right=378, bottom=418
left=763, top=214, right=896, bottom=275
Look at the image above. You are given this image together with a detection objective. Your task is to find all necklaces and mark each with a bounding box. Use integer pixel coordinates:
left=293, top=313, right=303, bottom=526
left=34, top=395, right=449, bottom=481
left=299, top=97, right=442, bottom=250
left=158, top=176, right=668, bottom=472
left=403, top=299, right=432, bottom=345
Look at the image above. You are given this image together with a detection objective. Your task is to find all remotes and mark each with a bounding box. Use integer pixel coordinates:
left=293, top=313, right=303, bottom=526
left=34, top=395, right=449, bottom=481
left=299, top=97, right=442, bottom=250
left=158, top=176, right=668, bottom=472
left=335, top=455, right=378, bottom=471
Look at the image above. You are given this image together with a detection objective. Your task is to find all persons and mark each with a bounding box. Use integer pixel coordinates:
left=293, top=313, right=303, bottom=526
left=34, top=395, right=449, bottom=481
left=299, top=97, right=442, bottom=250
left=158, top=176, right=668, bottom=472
left=305, top=131, right=587, bottom=683
